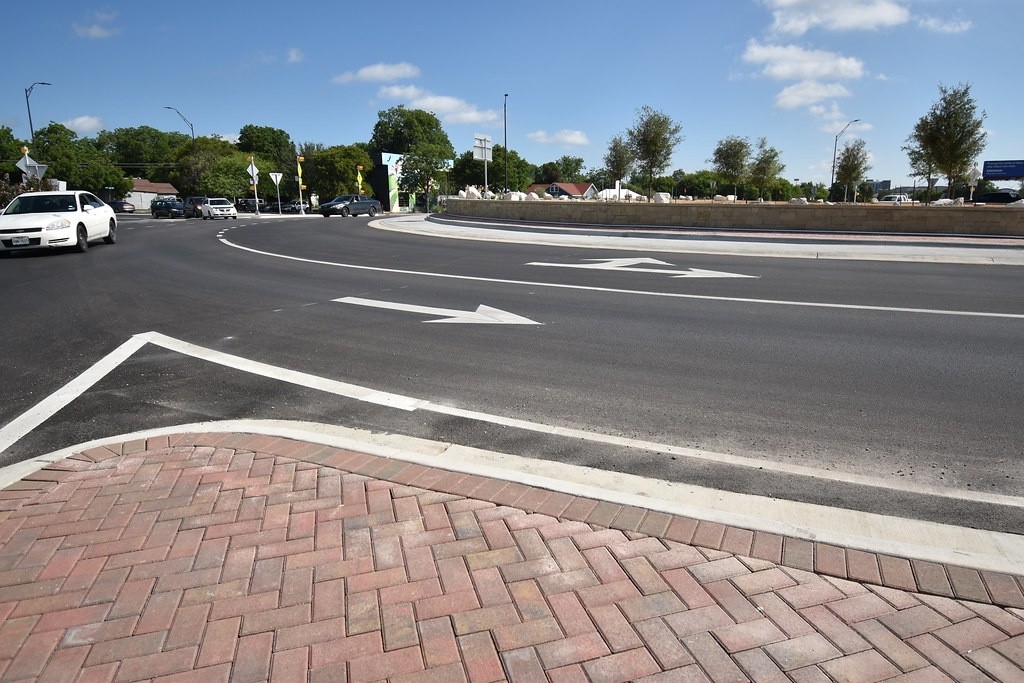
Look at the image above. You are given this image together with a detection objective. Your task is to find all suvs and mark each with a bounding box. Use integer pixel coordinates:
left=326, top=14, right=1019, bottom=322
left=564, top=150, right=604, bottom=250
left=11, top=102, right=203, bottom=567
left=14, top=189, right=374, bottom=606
left=964, top=190, right=1021, bottom=203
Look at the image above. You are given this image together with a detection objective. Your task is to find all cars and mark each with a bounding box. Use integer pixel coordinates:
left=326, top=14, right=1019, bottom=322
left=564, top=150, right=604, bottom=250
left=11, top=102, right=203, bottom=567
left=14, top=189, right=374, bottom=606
left=105, top=201, right=135, bottom=213
left=201, top=197, right=237, bottom=219
left=183, top=197, right=205, bottom=219
left=149, top=197, right=185, bottom=219
left=235, top=197, right=309, bottom=214
left=882, top=194, right=908, bottom=202
left=318, top=194, right=381, bottom=218
left=0, top=190, right=118, bottom=258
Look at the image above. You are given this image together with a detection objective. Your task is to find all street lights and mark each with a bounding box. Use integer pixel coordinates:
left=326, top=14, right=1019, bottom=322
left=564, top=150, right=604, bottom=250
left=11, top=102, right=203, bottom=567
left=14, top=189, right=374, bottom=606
left=794, top=178, right=799, bottom=187
left=504, top=93, right=510, bottom=191
left=25, top=81, right=53, bottom=165
left=830, top=119, right=862, bottom=203
left=165, top=107, right=194, bottom=147
